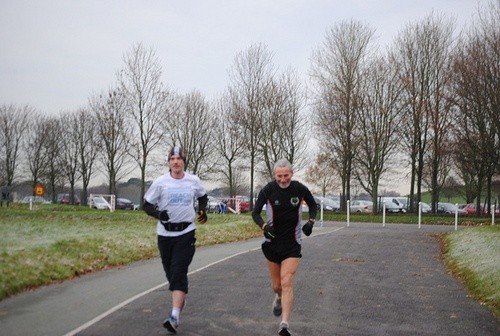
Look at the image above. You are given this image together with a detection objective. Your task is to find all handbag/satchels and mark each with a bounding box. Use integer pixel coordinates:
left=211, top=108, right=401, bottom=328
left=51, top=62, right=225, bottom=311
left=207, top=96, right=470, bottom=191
left=160, top=220, right=192, bottom=232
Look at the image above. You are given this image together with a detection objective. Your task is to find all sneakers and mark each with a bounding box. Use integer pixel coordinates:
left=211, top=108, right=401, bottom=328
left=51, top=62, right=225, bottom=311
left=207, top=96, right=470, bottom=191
left=278, top=322, right=292, bottom=336
left=162, top=316, right=179, bottom=334
left=272, top=294, right=282, bottom=317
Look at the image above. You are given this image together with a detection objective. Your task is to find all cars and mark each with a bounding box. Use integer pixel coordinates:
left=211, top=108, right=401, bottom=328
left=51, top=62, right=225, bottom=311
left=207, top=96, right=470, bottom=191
left=115, top=198, right=133, bottom=210
left=194, top=196, right=267, bottom=214
left=437, top=201, right=466, bottom=215
left=302, top=196, right=341, bottom=213
left=16, top=195, right=51, bottom=204
left=462, top=203, right=499, bottom=215
left=56, top=194, right=81, bottom=206
left=350, top=201, right=374, bottom=215
left=420, top=202, right=432, bottom=215
left=87, top=196, right=108, bottom=210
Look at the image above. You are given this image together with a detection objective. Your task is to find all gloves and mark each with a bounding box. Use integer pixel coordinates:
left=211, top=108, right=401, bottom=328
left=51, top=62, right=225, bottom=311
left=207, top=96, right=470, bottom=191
left=155, top=209, right=169, bottom=221
left=263, top=225, right=277, bottom=240
left=197, top=209, right=207, bottom=224
left=302, top=219, right=314, bottom=236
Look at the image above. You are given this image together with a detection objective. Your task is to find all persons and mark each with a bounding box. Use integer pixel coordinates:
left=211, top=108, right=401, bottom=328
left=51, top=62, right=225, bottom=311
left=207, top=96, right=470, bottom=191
left=252, top=158, right=316, bottom=336
left=143, top=146, right=210, bottom=333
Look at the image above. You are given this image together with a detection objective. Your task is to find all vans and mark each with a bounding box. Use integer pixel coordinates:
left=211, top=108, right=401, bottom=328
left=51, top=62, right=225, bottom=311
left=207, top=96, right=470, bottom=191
left=376, top=196, right=410, bottom=213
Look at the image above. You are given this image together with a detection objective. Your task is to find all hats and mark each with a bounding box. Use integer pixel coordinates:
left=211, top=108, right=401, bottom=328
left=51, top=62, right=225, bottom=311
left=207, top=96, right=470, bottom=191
left=167, top=146, right=186, bottom=162
